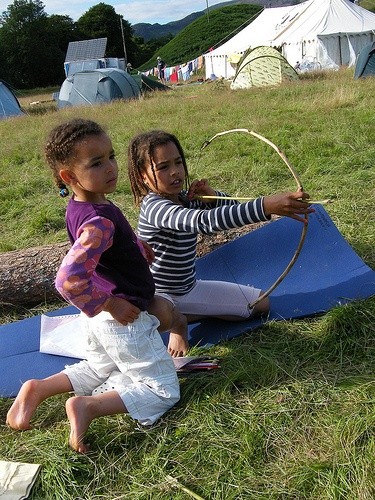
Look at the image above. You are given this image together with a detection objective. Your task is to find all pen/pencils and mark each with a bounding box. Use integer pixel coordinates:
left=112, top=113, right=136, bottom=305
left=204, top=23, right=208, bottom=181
left=176, top=360, right=222, bottom=376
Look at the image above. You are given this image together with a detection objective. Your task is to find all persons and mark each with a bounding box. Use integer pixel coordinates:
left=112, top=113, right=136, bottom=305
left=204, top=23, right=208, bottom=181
left=128, top=130, right=315, bottom=359
left=156, top=56, right=166, bottom=84
left=5, top=120, right=181, bottom=453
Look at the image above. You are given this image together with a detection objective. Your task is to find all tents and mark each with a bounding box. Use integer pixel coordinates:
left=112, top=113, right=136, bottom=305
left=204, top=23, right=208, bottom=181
left=0, top=81, right=24, bottom=117
left=57, top=68, right=141, bottom=108
left=203, top=0, right=375, bottom=91
